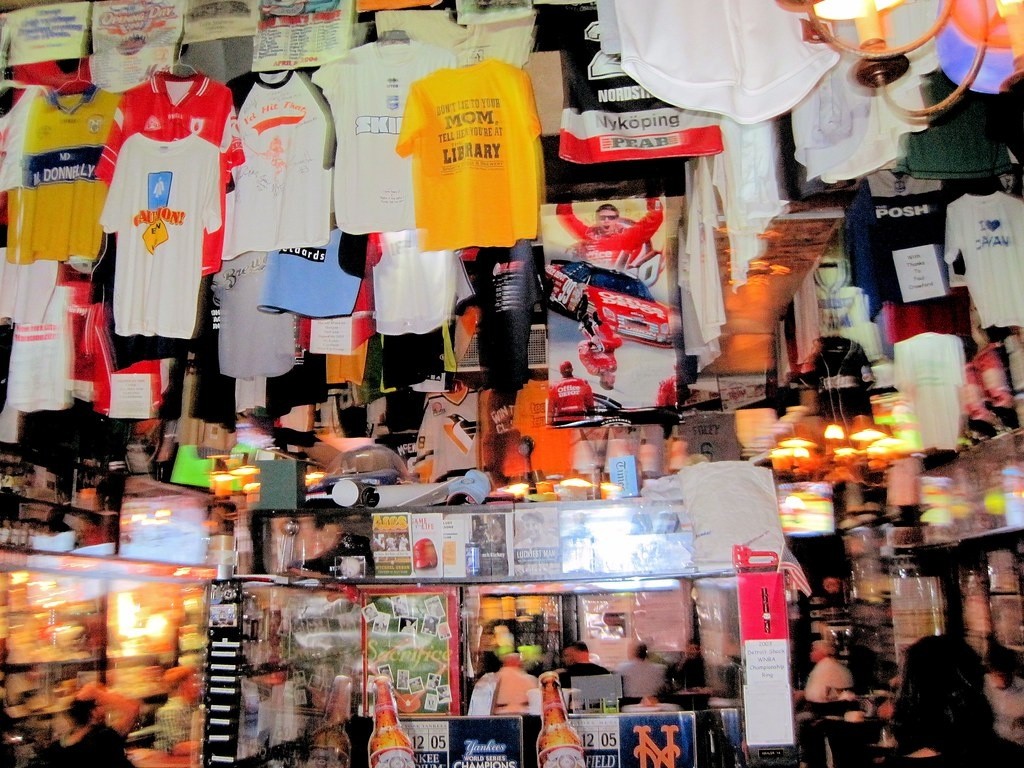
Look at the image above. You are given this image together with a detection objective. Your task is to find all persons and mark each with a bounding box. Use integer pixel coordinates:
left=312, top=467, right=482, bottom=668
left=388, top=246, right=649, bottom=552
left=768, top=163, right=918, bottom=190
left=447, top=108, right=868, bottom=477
left=804, top=641, right=854, bottom=767
left=493, top=642, right=611, bottom=714
left=548, top=197, right=678, bottom=423
left=515, top=511, right=559, bottom=576
left=674, top=638, right=708, bottom=710
left=616, top=642, right=668, bottom=705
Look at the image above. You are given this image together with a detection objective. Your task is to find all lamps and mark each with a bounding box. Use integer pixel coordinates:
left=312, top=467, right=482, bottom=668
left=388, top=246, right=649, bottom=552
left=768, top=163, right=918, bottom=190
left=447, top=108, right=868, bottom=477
left=775, top=0, right=1024, bottom=120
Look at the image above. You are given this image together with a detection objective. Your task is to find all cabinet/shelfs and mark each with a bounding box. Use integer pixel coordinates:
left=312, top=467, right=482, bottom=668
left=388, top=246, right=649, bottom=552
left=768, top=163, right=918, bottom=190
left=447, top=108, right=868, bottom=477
left=0, top=540, right=235, bottom=768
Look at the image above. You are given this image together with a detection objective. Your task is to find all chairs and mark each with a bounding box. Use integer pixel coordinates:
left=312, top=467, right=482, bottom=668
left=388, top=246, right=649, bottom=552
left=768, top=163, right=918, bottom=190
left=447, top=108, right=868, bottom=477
left=571, top=672, right=624, bottom=713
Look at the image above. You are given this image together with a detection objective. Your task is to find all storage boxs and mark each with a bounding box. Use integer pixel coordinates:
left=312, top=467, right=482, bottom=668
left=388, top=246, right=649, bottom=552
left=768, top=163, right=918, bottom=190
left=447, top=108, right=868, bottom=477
left=891, top=245, right=959, bottom=304
left=538, top=192, right=699, bottom=431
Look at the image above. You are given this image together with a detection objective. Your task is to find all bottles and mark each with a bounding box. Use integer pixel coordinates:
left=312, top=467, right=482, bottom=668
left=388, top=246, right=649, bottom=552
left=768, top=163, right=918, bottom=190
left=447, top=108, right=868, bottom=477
left=368, top=673, right=415, bottom=768
left=303, top=675, right=352, bottom=768
left=537, top=671, right=586, bottom=768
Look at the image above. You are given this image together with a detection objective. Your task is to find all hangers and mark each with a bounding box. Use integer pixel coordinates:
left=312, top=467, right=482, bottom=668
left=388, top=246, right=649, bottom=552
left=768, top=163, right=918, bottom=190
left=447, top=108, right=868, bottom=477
left=0, top=10, right=536, bottom=124
